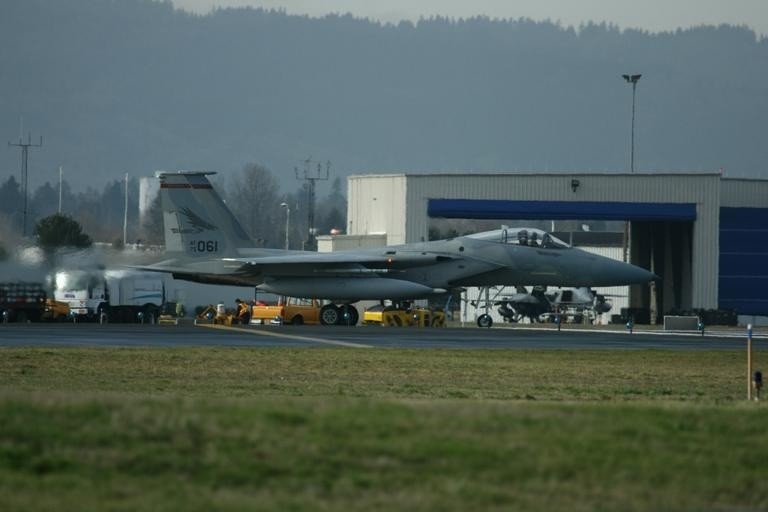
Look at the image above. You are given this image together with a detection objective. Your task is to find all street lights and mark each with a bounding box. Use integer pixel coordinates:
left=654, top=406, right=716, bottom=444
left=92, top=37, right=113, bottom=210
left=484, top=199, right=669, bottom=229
left=621, top=72, right=641, bottom=174
left=279, top=203, right=289, bottom=249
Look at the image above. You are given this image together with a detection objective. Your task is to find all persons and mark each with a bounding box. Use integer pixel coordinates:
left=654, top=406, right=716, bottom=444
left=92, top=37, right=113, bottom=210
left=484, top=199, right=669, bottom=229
left=698, top=320, right=705, bottom=337
left=132, top=239, right=141, bottom=250
left=555, top=315, right=561, bottom=332
left=2, top=309, right=9, bottom=326
left=626, top=316, right=633, bottom=337
left=235, top=298, right=251, bottom=325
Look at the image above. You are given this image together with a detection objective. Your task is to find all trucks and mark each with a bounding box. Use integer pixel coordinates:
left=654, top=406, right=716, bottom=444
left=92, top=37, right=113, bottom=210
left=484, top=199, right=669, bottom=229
left=0, top=282, right=46, bottom=324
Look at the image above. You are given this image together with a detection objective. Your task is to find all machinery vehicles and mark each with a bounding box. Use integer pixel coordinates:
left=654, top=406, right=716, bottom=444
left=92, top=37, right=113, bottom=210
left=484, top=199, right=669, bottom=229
left=250, top=288, right=326, bottom=325
left=48, top=268, right=166, bottom=325
left=363, top=296, right=446, bottom=328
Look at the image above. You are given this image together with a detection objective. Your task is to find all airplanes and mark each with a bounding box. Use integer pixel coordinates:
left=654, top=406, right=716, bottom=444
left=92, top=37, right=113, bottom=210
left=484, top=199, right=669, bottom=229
left=121, top=169, right=662, bottom=328
left=464, top=286, right=630, bottom=328
